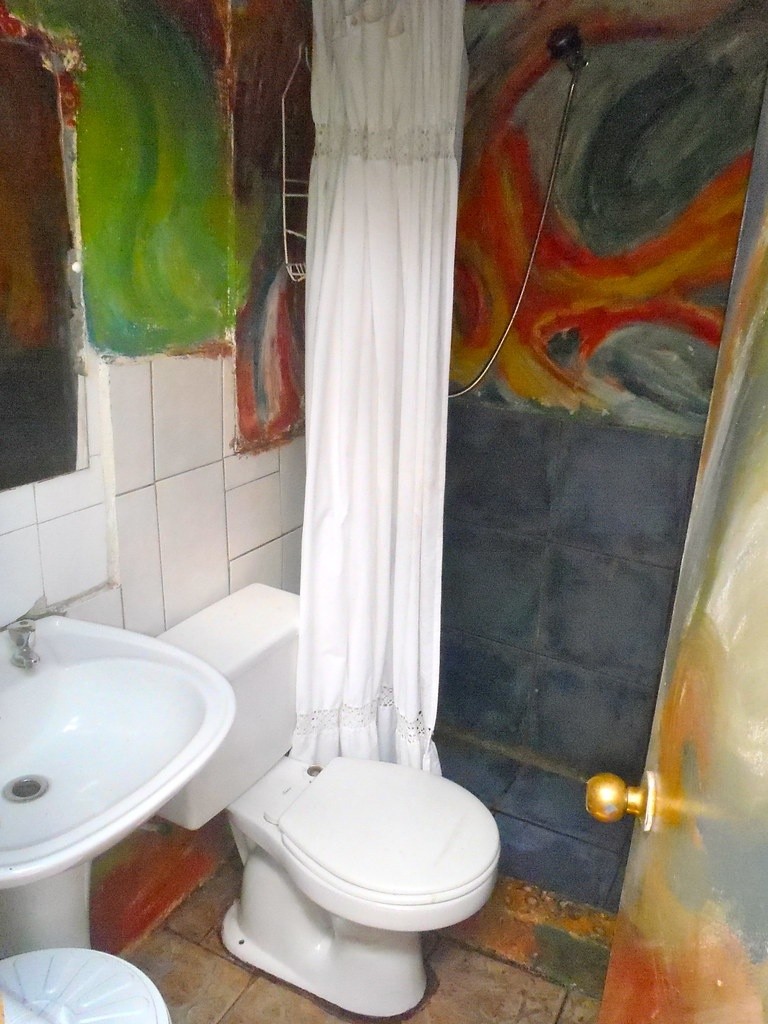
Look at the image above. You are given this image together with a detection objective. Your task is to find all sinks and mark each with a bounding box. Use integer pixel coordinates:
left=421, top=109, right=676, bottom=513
left=0, top=607, right=239, bottom=893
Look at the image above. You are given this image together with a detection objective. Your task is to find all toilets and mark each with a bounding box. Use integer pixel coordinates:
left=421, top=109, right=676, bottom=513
left=147, top=582, right=504, bottom=1017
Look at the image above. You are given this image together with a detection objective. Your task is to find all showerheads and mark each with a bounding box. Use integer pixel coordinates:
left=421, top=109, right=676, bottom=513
left=545, top=11, right=588, bottom=72
